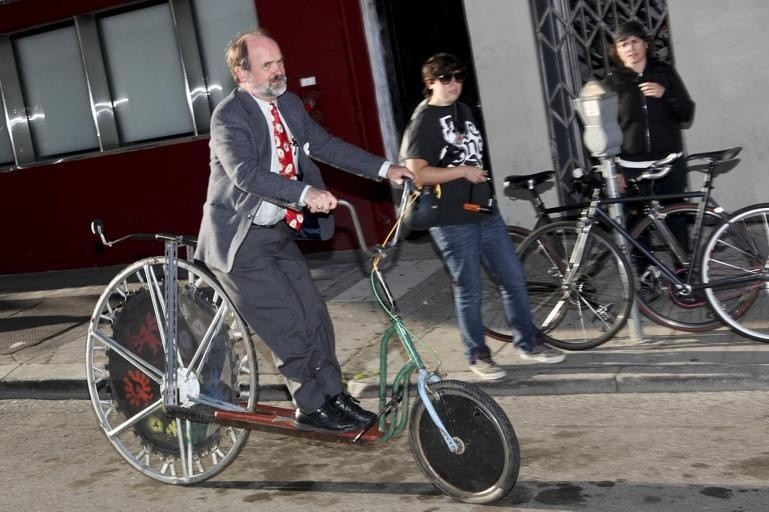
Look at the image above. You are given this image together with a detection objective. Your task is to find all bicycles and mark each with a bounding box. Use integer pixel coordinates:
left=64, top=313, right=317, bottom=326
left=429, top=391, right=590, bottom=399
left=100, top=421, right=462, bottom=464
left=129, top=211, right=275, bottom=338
left=83, top=176, right=519, bottom=504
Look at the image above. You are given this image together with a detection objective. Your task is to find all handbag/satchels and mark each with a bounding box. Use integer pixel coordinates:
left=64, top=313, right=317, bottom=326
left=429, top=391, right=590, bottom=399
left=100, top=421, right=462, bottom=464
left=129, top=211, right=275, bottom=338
left=400, top=171, right=497, bottom=234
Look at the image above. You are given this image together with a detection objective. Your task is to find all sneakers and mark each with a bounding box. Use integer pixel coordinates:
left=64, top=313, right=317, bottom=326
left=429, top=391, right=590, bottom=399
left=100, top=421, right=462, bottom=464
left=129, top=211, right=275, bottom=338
left=468, top=349, right=506, bottom=381
left=638, top=286, right=665, bottom=307
left=518, top=344, right=567, bottom=364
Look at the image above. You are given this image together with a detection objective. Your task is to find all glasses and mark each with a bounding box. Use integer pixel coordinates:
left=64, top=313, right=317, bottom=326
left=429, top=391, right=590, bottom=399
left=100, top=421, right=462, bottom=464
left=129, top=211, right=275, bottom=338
left=434, top=71, right=467, bottom=86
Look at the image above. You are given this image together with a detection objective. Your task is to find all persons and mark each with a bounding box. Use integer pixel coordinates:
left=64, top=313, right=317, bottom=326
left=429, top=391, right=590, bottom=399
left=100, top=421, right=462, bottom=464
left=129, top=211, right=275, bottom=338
left=398, top=52, right=569, bottom=381
left=603, top=19, right=698, bottom=306
left=191, top=28, right=419, bottom=437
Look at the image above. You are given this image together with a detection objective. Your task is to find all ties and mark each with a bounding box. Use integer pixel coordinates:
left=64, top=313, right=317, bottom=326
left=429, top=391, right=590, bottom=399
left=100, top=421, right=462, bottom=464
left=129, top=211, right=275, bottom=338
left=266, top=101, right=305, bottom=234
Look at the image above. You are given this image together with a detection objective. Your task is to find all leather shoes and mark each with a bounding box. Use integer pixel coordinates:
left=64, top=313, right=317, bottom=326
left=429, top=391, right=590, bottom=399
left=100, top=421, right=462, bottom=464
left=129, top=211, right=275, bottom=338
left=295, top=398, right=359, bottom=432
left=323, top=392, right=380, bottom=430
